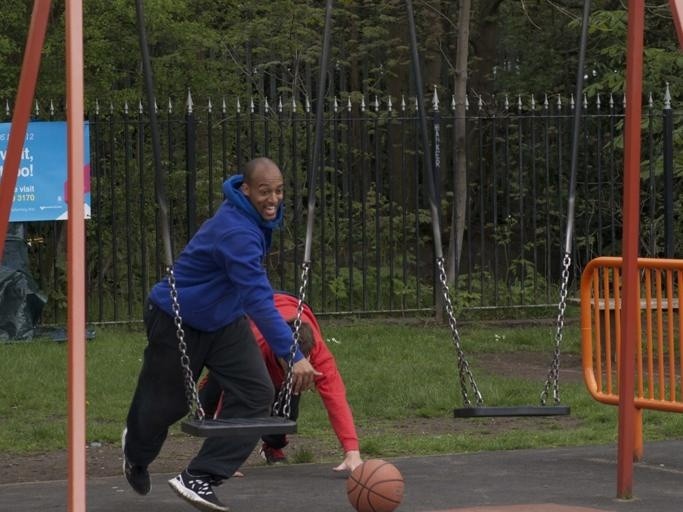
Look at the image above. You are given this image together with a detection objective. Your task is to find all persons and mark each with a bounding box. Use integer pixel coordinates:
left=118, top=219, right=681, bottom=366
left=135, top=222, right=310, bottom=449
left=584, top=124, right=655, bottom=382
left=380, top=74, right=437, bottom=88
left=119, top=156, right=324, bottom=512
left=199, top=290, right=365, bottom=475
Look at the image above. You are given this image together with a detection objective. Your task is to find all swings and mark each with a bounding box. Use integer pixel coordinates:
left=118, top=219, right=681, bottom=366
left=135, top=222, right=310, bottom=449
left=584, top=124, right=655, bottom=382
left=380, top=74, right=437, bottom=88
left=410, top=2, right=589, bottom=419
left=138, top=3, right=331, bottom=436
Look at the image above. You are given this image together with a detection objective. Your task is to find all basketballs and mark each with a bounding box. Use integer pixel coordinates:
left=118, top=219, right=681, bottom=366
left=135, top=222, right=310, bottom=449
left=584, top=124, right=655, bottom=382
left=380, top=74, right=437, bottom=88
left=347, top=458, right=405, bottom=511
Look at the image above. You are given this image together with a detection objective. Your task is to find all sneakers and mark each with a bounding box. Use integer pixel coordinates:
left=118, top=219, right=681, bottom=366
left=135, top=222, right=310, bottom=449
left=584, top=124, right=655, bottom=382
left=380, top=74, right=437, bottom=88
left=258, top=442, right=290, bottom=466
left=197, top=378, right=213, bottom=420
left=167, top=464, right=231, bottom=512
left=121, top=427, right=152, bottom=498
